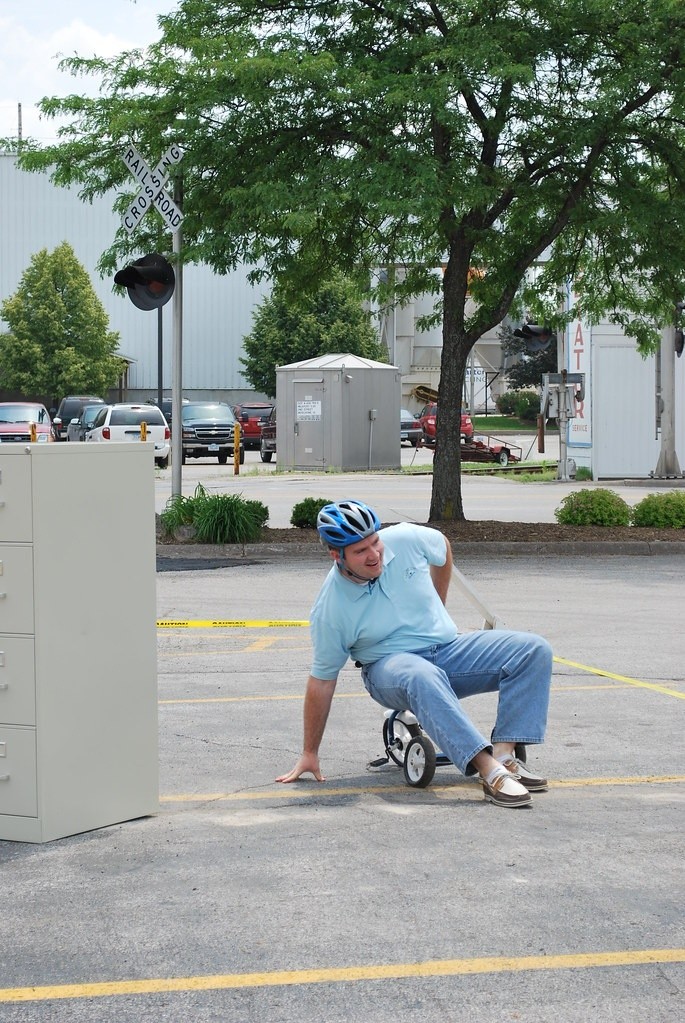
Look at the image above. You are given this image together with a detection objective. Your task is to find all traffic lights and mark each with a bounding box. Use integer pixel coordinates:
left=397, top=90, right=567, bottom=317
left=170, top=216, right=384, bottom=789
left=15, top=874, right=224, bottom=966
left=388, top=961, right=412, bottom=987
left=115, top=250, right=176, bottom=313
left=513, top=322, right=555, bottom=352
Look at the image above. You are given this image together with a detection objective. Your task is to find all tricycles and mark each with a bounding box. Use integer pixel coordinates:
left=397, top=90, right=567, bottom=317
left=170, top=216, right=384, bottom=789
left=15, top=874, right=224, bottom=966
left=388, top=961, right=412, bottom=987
left=368, top=707, right=526, bottom=789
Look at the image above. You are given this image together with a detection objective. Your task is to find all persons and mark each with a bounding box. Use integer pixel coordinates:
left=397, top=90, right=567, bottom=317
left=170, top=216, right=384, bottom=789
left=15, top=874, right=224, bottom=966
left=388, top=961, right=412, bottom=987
left=275, top=499, right=554, bottom=808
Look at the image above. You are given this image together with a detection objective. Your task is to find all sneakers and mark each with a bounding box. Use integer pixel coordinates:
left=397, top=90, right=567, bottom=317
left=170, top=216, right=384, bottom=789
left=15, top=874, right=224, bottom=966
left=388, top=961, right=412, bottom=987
left=477, top=767, right=532, bottom=808
left=502, top=758, right=548, bottom=791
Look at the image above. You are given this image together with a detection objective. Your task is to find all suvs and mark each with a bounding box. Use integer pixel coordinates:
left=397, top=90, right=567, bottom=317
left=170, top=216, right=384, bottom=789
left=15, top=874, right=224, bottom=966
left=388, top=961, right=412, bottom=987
left=82, top=402, right=170, bottom=469
left=52, top=394, right=105, bottom=443
left=230, top=401, right=275, bottom=449
left=182, top=401, right=245, bottom=465
left=414, top=401, right=474, bottom=444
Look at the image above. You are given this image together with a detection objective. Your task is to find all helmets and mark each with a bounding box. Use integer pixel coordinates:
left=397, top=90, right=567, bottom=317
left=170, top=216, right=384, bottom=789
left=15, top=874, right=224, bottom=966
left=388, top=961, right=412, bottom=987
left=316, top=499, right=381, bottom=549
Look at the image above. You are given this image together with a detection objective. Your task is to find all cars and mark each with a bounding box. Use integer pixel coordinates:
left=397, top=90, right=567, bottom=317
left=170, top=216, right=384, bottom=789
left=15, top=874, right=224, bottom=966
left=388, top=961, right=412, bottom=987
left=66, top=404, right=108, bottom=441
left=0, top=400, right=55, bottom=443
left=256, top=404, right=277, bottom=462
left=146, top=396, right=190, bottom=437
left=400, top=409, right=421, bottom=450
